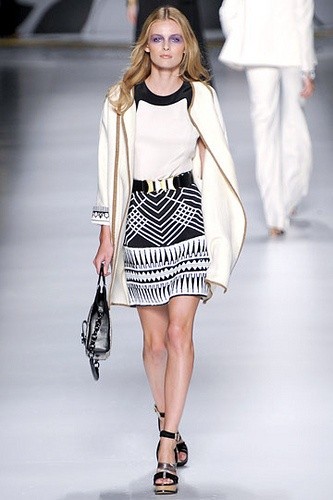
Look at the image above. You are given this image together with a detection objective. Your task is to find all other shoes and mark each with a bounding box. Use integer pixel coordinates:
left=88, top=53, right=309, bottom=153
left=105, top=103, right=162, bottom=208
left=270, top=227, right=286, bottom=237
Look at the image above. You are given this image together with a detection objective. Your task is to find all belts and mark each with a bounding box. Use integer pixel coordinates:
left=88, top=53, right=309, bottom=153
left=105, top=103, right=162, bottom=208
left=133, top=171, right=195, bottom=194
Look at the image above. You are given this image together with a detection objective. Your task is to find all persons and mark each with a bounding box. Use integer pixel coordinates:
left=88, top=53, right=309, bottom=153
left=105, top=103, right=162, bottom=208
left=217, top=0, right=317, bottom=236
left=93, top=7, right=248, bottom=495
left=126, top=0, right=214, bottom=89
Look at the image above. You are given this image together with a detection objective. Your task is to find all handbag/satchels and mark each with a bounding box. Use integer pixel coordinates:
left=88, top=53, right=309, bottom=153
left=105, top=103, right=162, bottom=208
left=81, top=263, right=112, bottom=382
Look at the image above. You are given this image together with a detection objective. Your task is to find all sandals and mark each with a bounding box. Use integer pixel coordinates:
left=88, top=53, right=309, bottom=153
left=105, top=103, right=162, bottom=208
left=158, top=414, right=189, bottom=465
left=152, top=432, right=179, bottom=493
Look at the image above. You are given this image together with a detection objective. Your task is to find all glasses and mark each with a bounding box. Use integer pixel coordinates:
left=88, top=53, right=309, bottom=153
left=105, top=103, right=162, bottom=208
left=149, top=34, right=185, bottom=45
